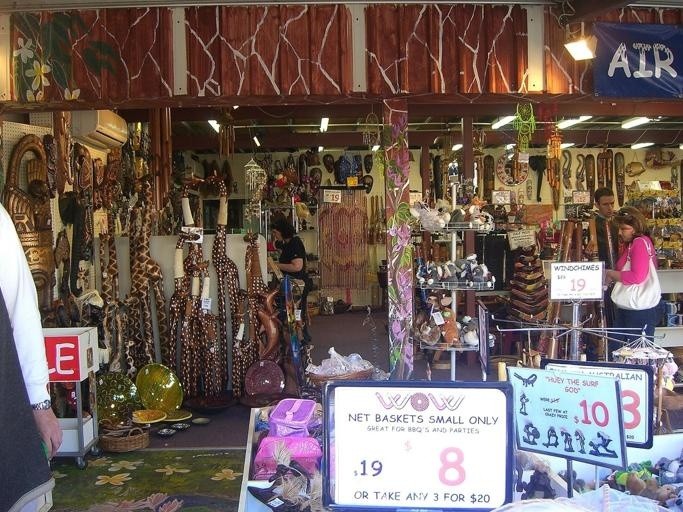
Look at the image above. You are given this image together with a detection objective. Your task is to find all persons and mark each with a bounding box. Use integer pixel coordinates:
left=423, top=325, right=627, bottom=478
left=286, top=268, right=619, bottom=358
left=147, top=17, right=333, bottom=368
left=0, top=200, right=63, bottom=512
left=267, top=213, right=312, bottom=350
left=588, top=186, right=663, bottom=363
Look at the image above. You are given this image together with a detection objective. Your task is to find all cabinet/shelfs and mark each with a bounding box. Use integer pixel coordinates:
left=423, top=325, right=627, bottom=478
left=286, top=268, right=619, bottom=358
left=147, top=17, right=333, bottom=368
left=234, top=407, right=682, bottom=512
left=651, top=268, right=683, bottom=409
left=416, top=185, right=495, bottom=381
left=258, top=198, right=322, bottom=315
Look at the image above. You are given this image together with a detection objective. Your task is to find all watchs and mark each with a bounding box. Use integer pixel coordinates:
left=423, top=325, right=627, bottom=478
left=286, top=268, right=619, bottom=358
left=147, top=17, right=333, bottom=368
left=29, top=400, right=54, bottom=411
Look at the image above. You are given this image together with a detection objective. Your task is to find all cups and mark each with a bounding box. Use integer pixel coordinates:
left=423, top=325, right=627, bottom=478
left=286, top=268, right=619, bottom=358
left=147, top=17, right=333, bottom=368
left=664, top=301, right=683, bottom=327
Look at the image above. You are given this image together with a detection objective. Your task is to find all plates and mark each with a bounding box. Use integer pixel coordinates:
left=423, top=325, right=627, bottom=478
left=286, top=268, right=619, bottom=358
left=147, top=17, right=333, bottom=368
left=96, top=360, right=296, bottom=429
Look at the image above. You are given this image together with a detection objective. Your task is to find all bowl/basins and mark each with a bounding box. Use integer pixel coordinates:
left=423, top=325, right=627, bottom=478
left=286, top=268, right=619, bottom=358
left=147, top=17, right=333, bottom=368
left=158, top=428, right=176, bottom=438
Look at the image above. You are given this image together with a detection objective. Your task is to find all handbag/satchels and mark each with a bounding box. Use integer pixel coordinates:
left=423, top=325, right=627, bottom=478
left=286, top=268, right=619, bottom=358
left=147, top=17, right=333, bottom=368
left=610, top=258, right=661, bottom=311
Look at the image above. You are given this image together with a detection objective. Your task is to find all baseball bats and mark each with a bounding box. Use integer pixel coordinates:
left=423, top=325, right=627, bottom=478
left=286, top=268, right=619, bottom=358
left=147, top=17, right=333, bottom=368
left=536, top=215, right=608, bottom=363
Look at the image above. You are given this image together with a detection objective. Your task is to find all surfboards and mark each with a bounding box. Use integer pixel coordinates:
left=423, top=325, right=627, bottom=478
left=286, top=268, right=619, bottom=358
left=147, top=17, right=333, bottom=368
left=284, top=274, right=306, bottom=398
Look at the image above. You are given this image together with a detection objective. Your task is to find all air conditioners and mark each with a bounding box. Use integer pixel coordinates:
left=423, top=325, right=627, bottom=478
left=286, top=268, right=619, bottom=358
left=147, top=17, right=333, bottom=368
left=72, top=110, right=128, bottom=149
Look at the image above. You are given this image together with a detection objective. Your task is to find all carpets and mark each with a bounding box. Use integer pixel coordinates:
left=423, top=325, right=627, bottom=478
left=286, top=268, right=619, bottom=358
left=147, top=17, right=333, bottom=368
left=48, top=447, right=245, bottom=511
